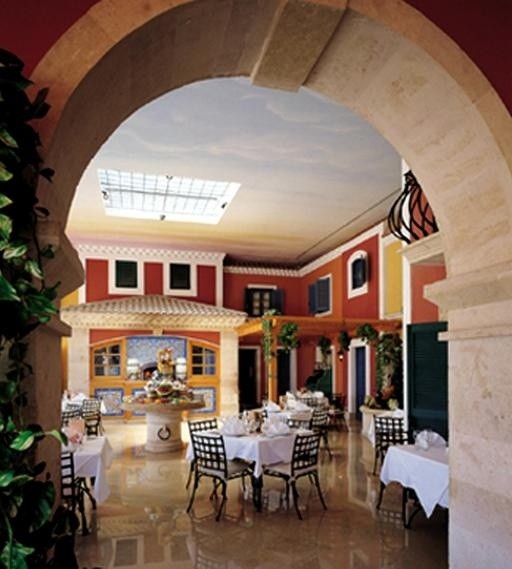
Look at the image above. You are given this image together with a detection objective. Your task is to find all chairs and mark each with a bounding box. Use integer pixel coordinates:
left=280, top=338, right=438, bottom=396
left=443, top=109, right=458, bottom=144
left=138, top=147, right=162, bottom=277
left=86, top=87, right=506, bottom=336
left=185, top=392, right=349, bottom=522
left=373, top=414, right=419, bottom=530
left=60, top=399, right=106, bottom=537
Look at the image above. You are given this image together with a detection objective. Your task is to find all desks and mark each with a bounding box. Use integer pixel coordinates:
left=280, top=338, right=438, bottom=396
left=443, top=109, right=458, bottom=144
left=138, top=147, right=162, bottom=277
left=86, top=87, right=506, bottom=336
left=118, top=401, right=206, bottom=454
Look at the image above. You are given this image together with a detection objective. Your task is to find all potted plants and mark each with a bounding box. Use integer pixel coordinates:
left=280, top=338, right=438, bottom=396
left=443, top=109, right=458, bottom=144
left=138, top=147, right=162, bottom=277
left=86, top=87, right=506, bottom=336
left=359, top=333, right=404, bottom=447
